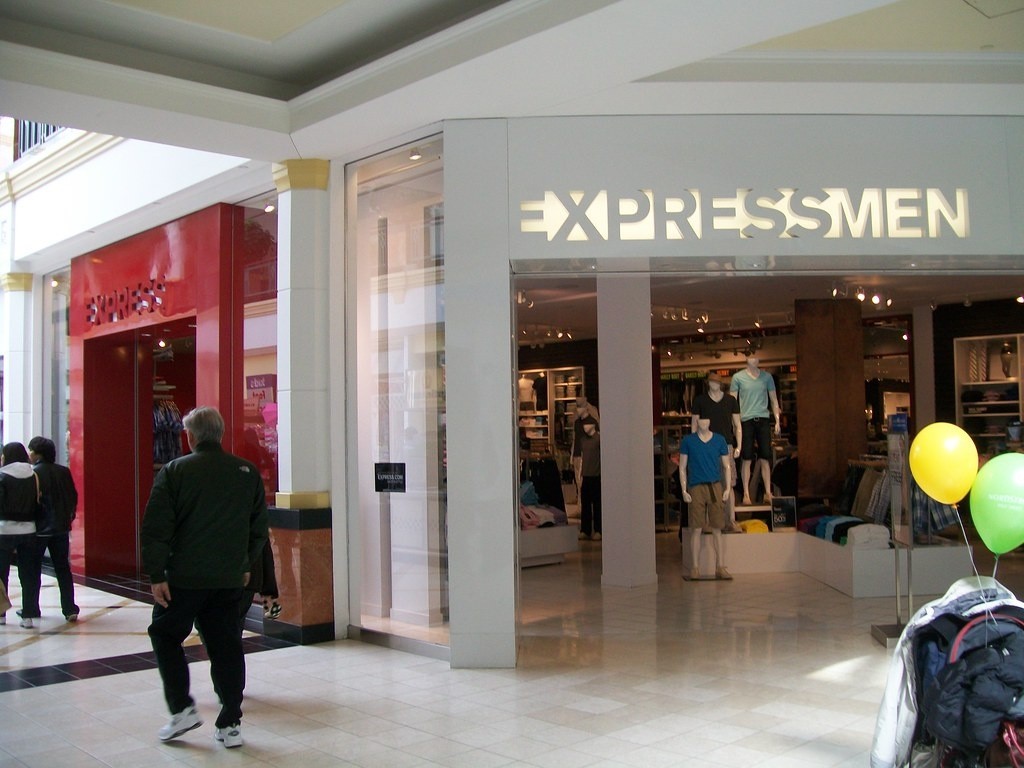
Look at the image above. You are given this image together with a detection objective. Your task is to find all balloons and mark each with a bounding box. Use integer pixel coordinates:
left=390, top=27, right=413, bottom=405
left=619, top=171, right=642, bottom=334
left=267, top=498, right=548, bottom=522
left=909, top=422, right=979, bottom=509
left=970, top=452, right=1024, bottom=560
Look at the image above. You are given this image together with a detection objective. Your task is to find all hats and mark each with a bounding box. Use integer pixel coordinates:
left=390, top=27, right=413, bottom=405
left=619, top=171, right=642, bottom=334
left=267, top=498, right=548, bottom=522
left=704, top=373, right=724, bottom=385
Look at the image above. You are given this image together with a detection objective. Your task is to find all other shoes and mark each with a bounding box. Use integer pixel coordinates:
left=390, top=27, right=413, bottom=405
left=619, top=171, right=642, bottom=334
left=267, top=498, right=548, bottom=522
left=578, top=532, right=590, bottom=540
left=16, top=609, right=41, bottom=618
left=690, top=567, right=701, bottom=580
left=727, top=520, right=743, bottom=533
left=65, top=613, right=78, bottom=622
left=762, top=493, right=774, bottom=505
left=742, top=497, right=753, bottom=506
left=0, top=615, right=7, bottom=624
left=592, top=532, right=602, bottom=541
left=715, top=566, right=733, bottom=580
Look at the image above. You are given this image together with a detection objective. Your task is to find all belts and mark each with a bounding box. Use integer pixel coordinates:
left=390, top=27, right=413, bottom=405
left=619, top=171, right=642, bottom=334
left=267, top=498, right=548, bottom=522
left=707, top=482, right=717, bottom=503
left=750, top=417, right=769, bottom=422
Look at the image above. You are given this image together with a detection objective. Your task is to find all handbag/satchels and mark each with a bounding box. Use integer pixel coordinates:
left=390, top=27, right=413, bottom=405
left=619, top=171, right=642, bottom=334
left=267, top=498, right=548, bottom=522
left=1, top=581, right=13, bottom=615
left=36, top=491, right=50, bottom=532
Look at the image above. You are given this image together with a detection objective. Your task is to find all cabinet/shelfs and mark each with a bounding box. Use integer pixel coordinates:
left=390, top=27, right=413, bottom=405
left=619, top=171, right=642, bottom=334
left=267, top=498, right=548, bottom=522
left=953, top=335, right=1024, bottom=439
left=517, top=365, right=585, bottom=451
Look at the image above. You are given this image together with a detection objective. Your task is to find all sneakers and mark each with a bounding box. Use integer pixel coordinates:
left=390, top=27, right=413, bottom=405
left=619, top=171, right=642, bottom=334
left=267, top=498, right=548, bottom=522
left=215, top=723, right=243, bottom=748
left=156, top=705, right=204, bottom=743
left=19, top=618, right=34, bottom=628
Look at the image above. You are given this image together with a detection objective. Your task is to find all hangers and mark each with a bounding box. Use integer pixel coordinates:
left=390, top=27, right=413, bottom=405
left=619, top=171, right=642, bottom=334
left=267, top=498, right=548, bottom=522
left=153, top=395, right=178, bottom=403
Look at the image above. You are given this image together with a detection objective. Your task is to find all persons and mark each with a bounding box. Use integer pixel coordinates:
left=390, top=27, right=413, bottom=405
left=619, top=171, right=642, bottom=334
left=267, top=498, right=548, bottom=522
left=571, top=391, right=602, bottom=540
left=691, top=358, right=781, bottom=533
left=517, top=373, right=547, bottom=411
left=679, top=419, right=732, bottom=580
left=0, top=436, right=80, bottom=628
left=139, top=405, right=278, bottom=749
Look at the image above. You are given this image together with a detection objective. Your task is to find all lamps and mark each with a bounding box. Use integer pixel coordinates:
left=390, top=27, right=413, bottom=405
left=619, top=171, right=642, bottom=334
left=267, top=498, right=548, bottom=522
left=566, top=330, right=576, bottom=339
left=964, top=294, right=972, bottom=308
left=556, top=329, right=563, bottom=339
left=522, top=297, right=534, bottom=308
left=649, top=306, right=766, bottom=359
